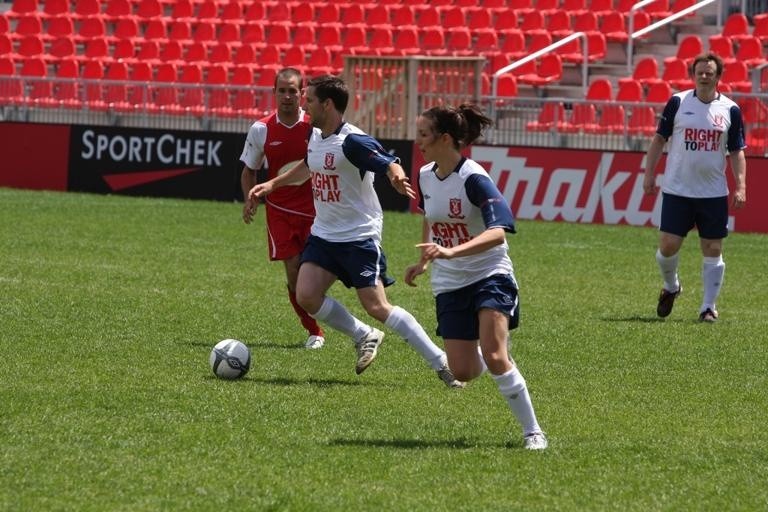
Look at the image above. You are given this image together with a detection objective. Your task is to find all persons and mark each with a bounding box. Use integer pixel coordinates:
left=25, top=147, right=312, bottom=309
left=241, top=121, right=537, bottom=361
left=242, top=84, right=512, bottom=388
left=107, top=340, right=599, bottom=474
left=404, top=102, right=547, bottom=450
left=240, top=69, right=324, bottom=350
left=643, top=53, right=747, bottom=322
left=248, top=74, right=464, bottom=386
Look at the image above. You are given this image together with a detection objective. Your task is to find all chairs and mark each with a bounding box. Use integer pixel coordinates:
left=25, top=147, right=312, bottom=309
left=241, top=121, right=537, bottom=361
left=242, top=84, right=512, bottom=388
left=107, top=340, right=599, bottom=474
left=526, top=12, right=768, bottom=157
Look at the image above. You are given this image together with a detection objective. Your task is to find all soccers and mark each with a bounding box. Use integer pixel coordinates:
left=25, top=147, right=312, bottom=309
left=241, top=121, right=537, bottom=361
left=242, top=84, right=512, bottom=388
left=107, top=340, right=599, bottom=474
left=209, top=339, right=250, bottom=379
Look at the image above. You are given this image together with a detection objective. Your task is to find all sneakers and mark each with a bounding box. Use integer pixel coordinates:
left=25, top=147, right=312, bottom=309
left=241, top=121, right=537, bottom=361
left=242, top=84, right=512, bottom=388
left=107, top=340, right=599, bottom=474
left=521, top=430, right=548, bottom=452
left=657, top=281, right=683, bottom=317
left=436, top=361, right=466, bottom=389
left=698, top=305, right=720, bottom=323
left=305, top=335, right=325, bottom=351
left=354, top=323, right=385, bottom=375
